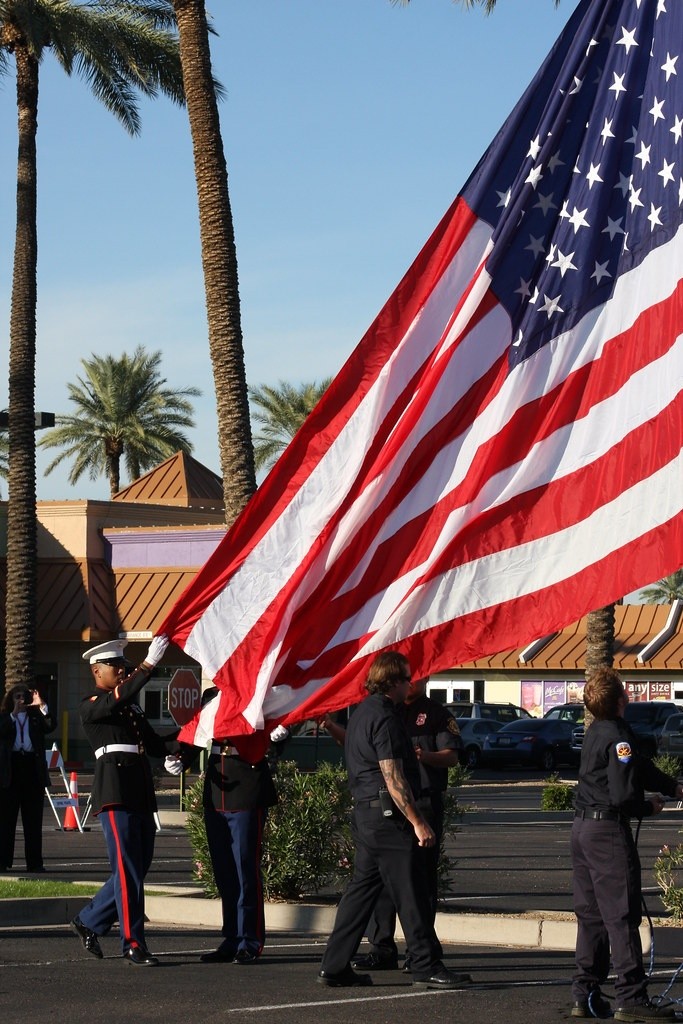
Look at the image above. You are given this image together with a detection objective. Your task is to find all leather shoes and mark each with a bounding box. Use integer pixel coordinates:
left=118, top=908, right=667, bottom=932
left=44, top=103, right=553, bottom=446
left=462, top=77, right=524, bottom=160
left=123, top=946, right=159, bottom=967
left=200, top=949, right=234, bottom=962
left=231, top=951, right=256, bottom=964
left=571, top=997, right=610, bottom=1018
left=614, top=1002, right=676, bottom=1023
left=352, top=952, right=399, bottom=970
left=69, top=915, right=103, bottom=959
left=316, top=970, right=373, bottom=987
left=411, top=968, right=471, bottom=990
left=402, top=955, right=412, bottom=973
left=94, top=743, right=144, bottom=759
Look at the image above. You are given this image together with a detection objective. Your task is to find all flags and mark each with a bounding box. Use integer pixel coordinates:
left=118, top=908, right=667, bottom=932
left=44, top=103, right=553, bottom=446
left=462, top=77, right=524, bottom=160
left=151, top=0, right=683, bottom=766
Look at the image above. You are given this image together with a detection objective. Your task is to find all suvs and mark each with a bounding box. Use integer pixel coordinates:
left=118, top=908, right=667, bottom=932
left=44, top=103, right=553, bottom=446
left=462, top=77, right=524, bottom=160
left=442, top=703, right=537, bottom=718
left=569, top=701, right=680, bottom=774
left=543, top=702, right=585, bottom=718
left=655, top=713, right=683, bottom=759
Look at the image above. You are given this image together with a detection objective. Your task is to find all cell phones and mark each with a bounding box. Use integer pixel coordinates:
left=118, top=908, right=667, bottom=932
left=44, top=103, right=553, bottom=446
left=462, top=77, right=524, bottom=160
left=24, top=690, right=32, bottom=704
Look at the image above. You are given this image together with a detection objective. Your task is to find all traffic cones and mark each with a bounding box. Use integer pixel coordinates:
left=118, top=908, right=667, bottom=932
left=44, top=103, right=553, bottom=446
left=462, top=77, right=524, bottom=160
left=63, top=772, right=80, bottom=828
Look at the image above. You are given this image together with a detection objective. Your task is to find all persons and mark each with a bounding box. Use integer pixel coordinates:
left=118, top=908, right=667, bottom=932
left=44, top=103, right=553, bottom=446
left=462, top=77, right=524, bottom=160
left=316, top=648, right=471, bottom=990
left=571, top=666, right=683, bottom=1024
left=0, top=685, right=58, bottom=872
left=71, top=633, right=169, bottom=966
left=163, top=685, right=290, bottom=964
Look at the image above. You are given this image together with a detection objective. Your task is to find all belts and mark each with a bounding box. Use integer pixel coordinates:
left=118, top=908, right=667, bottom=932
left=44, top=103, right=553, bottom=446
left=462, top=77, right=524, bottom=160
left=211, top=746, right=239, bottom=756
left=575, top=808, right=626, bottom=821
left=354, top=799, right=382, bottom=808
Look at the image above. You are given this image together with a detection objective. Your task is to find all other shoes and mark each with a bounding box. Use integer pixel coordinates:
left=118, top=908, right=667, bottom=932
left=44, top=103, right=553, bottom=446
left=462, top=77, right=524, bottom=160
left=27, top=866, right=45, bottom=873
left=0, top=866, right=6, bottom=872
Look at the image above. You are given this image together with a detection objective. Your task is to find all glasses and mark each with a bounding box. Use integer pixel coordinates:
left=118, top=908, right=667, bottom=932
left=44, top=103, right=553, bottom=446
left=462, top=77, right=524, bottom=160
left=15, top=694, right=24, bottom=699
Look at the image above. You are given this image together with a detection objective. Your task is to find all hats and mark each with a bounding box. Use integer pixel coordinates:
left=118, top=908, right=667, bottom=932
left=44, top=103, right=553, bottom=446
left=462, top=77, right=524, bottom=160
left=82, top=639, right=132, bottom=667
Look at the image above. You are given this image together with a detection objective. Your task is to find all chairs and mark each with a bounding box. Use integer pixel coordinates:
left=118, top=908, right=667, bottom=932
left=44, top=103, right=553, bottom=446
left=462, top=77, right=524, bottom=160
left=570, top=711, right=585, bottom=721
left=455, top=707, right=466, bottom=718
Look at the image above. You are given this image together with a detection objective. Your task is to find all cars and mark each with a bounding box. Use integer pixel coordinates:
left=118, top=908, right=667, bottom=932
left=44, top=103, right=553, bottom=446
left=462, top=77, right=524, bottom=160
left=454, top=718, right=506, bottom=772
left=481, top=718, right=576, bottom=772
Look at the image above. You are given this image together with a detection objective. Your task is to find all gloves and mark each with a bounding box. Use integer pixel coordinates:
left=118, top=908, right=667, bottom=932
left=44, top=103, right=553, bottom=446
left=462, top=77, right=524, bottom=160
left=144, top=633, right=169, bottom=667
left=163, top=754, right=183, bottom=776
left=269, top=724, right=288, bottom=742
